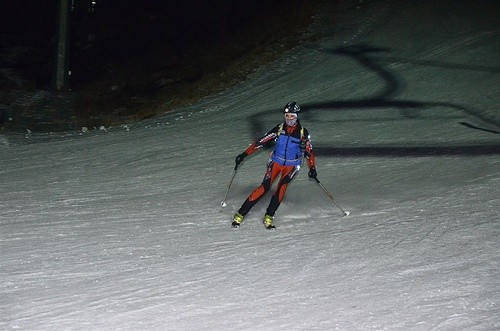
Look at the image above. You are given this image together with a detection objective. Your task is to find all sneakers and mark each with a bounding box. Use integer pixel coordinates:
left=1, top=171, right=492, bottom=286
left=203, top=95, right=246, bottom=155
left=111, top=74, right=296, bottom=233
left=232, top=212, right=244, bottom=229
left=262, top=215, right=276, bottom=232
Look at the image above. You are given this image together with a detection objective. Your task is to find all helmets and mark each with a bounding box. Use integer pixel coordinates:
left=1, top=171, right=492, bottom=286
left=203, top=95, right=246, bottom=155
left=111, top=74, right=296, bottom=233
left=283, top=101, right=301, bottom=113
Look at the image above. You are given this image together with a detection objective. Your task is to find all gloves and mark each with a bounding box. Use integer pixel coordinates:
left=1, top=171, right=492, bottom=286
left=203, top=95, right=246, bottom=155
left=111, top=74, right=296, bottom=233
left=235, top=151, right=247, bottom=167
left=308, top=166, right=318, bottom=179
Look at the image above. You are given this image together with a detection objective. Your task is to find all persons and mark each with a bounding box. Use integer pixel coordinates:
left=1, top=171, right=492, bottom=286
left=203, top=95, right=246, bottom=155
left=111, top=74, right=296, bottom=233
left=231, top=101, right=316, bottom=231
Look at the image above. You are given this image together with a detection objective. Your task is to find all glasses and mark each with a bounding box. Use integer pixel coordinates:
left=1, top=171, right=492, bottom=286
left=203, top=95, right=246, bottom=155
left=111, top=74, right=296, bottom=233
left=284, top=113, right=299, bottom=119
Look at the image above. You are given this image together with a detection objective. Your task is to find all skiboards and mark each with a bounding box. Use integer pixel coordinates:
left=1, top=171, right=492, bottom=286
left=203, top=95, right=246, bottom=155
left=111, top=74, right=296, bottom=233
left=230, top=206, right=277, bottom=230
